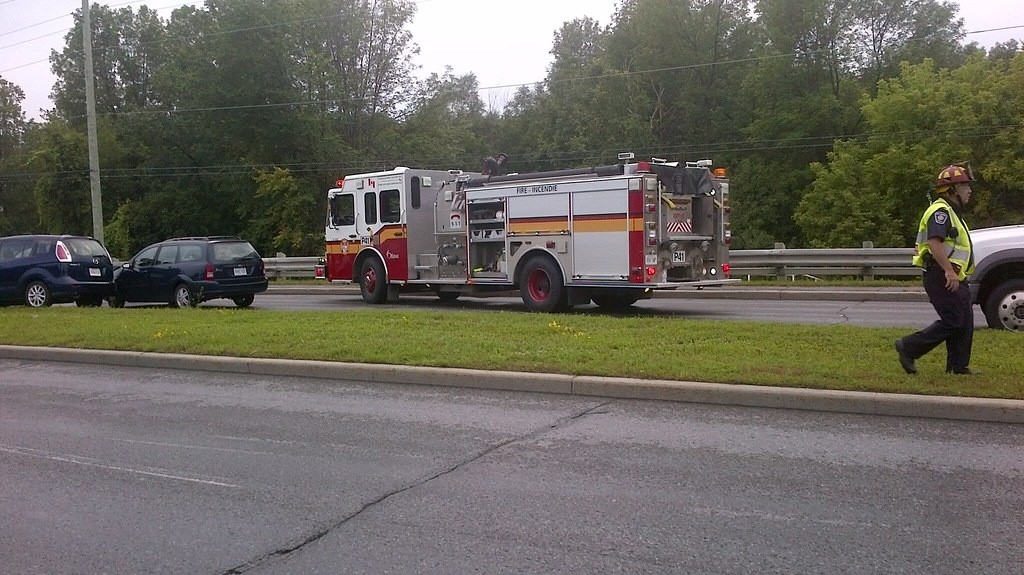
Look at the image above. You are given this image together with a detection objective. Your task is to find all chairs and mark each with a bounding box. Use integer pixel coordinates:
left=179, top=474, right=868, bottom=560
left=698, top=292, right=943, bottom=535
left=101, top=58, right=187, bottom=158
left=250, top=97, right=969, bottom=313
left=3, top=249, right=13, bottom=260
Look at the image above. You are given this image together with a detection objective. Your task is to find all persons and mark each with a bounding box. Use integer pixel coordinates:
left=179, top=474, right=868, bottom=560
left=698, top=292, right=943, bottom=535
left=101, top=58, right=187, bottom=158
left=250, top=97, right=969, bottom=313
left=895, top=165, right=981, bottom=375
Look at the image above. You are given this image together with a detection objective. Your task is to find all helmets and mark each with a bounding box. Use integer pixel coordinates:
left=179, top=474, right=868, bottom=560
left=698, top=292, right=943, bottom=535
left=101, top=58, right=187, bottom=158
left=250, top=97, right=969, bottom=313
left=933, top=165, right=974, bottom=192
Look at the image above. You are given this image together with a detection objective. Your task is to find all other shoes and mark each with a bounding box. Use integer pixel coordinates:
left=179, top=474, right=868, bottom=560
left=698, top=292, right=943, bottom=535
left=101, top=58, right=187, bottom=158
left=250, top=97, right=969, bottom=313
left=895, top=338, right=916, bottom=373
left=947, top=366, right=973, bottom=375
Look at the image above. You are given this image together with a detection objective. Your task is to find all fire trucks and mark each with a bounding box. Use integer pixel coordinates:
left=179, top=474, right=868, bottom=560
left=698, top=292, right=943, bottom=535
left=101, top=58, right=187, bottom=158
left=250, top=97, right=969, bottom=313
left=315, top=154, right=740, bottom=312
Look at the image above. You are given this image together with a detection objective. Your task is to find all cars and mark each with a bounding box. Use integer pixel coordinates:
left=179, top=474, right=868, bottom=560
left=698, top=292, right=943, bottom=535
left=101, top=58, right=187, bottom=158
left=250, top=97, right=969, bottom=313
left=0, top=235, right=116, bottom=307
left=930, top=225, right=1024, bottom=332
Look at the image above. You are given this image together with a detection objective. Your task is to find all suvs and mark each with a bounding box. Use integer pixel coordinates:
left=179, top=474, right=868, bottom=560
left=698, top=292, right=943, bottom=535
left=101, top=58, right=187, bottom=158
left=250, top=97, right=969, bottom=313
left=104, top=235, right=268, bottom=307
